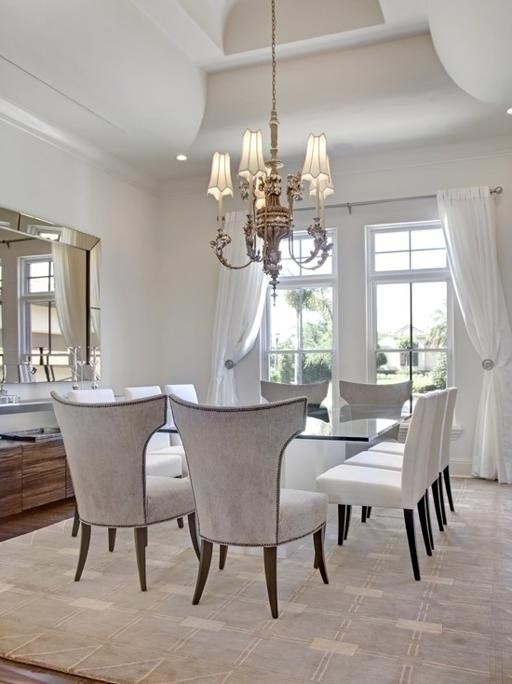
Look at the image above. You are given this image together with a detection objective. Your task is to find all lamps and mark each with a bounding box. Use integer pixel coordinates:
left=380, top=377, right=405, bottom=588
left=205, top=1, right=335, bottom=305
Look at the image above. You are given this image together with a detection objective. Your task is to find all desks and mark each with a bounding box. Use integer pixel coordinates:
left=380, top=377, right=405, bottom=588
left=156, top=415, right=402, bottom=570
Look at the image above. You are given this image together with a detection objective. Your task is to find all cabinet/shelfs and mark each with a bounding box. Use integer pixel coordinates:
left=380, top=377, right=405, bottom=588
left=0, top=430, right=75, bottom=518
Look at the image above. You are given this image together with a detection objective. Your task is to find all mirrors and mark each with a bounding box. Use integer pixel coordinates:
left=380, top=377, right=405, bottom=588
left=0, top=202, right=102, bottom=383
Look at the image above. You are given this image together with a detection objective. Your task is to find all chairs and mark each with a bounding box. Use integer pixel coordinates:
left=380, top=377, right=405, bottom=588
left=51, top=389, right=205, bottom=592
left=313, top=391, right=439, bottom=580
left=170, top=393, right=329, bottom=619
left=48, top=381, right=459, bottom=552
